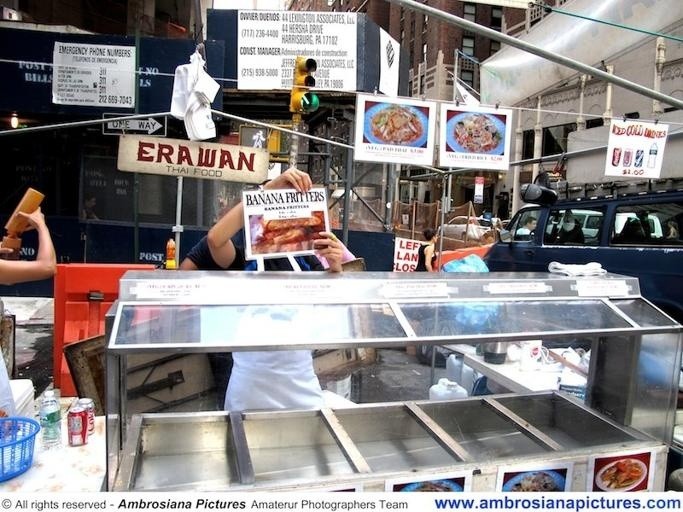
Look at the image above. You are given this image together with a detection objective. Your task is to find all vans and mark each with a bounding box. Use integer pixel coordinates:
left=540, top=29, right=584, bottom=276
left=483, top=187, right=683, bottom=325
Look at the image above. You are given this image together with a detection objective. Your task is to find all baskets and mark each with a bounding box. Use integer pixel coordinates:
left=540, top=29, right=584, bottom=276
left=0, top=414, right=40, bottom=482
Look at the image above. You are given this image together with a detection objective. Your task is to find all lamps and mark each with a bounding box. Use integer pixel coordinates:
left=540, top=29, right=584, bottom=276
left=519, top=168, right=565, bottom=208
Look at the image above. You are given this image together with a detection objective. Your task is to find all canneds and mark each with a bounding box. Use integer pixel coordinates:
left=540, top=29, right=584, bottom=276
left=623, top=149, right=632, bottom=167
left=67, top=407, right=89, bottom=447
left=78, top=398, right=96, bottom=436
left=634, top=149, right=644, bottom=168
left=612, top=148, right=621, bottom=166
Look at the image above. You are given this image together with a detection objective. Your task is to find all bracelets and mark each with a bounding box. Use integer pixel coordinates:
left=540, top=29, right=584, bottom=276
left=257, top=183, right=264, bottom=189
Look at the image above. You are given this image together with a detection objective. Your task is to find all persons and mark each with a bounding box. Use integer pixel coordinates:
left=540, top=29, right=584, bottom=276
left=410, top=228, right=439, bottom=273
left=0, top=206, right=57, bottom=419
left=83, top=190, right=104, bottom=219
left=178, top=228, right=245, bottom=410
left=515, top=215, right=538, bottom=236
left=205, top=167, right=343, bottom=413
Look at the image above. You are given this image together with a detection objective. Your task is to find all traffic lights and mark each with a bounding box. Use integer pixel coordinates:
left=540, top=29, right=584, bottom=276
left=289, top=56, right=320, bottom=115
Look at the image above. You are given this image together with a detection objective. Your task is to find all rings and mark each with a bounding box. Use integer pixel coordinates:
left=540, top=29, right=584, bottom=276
left=329, top=233, right=336, bottom=241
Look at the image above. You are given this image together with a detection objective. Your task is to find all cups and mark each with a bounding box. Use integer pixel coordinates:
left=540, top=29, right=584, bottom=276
left=1, top=238, right=23, bottom=261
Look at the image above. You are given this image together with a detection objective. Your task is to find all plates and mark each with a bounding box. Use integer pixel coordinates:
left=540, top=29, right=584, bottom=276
left=398, top=480, right=464, bottom=492
left=363, top=102, right=429, bottom=148
left=446, top=113, right=507, bottom=156
left=595, top=458, right=648, bottom=492
left=501, top=470, right=565, bottom=492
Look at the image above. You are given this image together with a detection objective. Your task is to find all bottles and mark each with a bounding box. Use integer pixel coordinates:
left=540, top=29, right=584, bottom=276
left=39, top=391, right=62, bottom=451
left=649, top=141, right=659, bottom=169
left=3, top=186, right=45, bottom=238
left=166, top=238, right=176, bottom=269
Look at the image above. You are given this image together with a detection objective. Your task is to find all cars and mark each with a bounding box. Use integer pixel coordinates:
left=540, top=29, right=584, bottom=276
left=437, top=216, right=511, bottom=241
